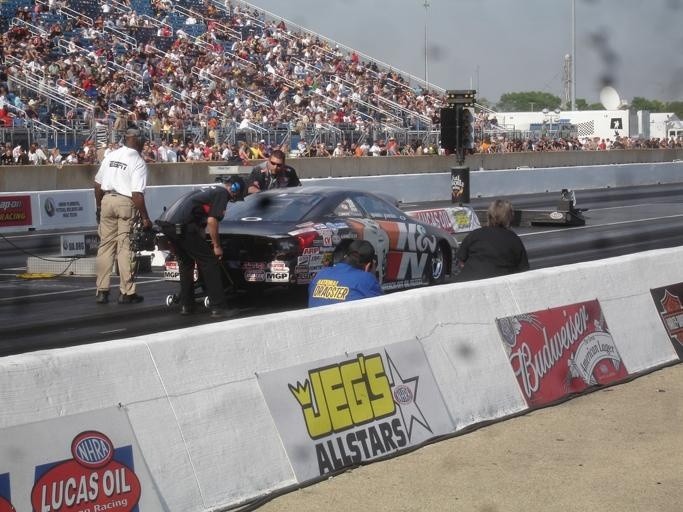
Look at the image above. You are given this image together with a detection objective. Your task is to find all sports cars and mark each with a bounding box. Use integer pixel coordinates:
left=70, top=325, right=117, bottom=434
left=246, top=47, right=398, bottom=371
left=162, top=186, right=465, bottom=295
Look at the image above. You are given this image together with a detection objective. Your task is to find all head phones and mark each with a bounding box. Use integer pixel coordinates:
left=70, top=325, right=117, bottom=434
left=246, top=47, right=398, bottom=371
left=225, top=176, right=240, bottom=193
left=122, top=125, right=145, bottom=145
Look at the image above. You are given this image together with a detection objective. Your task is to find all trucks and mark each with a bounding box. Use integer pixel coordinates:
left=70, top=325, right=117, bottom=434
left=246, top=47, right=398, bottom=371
left=650, top=114, right=683, bottom=144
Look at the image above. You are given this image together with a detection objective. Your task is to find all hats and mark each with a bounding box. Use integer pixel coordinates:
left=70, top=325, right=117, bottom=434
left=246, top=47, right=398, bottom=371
left=128, top=129, right=144, bottom=137
left=350, top=240, right=374, bottom=262
left=226, top=175, right=244, bottom=201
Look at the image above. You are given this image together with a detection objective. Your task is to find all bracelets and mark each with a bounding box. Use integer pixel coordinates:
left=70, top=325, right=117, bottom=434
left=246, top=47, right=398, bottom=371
left=143, top=219, right=149, bottom=221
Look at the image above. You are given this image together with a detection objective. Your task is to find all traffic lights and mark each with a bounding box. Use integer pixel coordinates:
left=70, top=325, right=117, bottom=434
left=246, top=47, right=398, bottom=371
left=460, top=107, right=475, bottom=148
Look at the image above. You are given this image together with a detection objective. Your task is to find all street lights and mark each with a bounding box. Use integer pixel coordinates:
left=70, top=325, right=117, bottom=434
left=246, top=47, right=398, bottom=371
left=421, top=0, right=431, bottom=94
left=543, top=107, right=561, bottom=136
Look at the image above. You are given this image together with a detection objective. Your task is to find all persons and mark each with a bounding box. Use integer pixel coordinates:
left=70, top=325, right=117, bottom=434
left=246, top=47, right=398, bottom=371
left=244, top=150, right=300, bottom=195
left=1, top=2, right=682, bottom=158
left=90, top=131, right=153, bottom=306
left=155, top=172, right=244, bottom=321
left=306, top=241, right=384, bottom=309
left=450, top=198, right=529, bottom=282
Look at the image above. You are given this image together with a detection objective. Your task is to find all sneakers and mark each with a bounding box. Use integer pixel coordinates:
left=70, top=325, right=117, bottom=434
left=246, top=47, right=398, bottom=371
left=118, top=293, right=144, bottom=303
left=211, top=308, right=240, bottom=318
left=96, top=291, right=109, bottom=304
left=180, top=304, right=202, bottom=314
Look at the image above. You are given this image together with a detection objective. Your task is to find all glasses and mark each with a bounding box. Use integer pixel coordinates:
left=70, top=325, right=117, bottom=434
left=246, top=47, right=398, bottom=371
left=271, top=162, right=283, bottom=167
